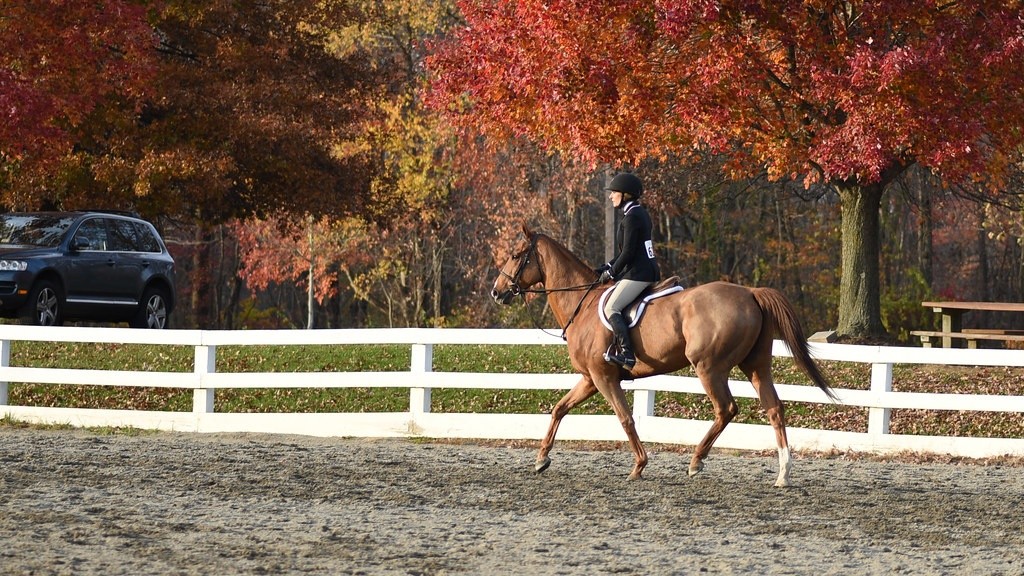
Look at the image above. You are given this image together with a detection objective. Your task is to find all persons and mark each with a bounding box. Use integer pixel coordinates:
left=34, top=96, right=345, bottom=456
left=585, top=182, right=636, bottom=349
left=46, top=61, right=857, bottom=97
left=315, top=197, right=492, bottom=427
left=594, top=172, right=662, bottom=371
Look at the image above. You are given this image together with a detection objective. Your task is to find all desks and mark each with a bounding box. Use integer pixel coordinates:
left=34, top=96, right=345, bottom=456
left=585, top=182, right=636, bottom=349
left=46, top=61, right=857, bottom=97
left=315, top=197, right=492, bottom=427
left=922, top=302, right=1024, bottom=350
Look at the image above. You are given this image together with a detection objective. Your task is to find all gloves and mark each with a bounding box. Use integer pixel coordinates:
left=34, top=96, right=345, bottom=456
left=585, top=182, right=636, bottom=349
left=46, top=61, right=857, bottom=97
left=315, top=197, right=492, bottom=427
left=595, top=264, right=610, bottom=272
left=598, top=270, right=610, bottom=285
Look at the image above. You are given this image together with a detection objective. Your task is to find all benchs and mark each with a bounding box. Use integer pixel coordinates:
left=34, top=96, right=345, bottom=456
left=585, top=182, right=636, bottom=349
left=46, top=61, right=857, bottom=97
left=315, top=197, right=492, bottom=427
left=910, top=330, right=1024, bottom=342
left=962, top=329, right=1024, bottom=335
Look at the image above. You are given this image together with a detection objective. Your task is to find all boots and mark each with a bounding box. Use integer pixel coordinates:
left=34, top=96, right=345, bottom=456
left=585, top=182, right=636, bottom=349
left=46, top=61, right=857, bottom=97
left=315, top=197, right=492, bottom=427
left=608, top=313, right=636, bottom=371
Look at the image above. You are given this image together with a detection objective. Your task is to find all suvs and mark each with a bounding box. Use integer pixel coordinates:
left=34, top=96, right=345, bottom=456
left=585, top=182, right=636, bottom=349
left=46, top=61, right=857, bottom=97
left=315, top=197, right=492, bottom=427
left=0, top=209, right=176, bottom=329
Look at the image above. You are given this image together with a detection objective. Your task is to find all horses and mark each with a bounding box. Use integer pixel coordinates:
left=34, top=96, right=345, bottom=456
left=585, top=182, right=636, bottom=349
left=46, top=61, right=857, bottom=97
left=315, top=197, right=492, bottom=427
left=490, top=218, right=844, bottom=489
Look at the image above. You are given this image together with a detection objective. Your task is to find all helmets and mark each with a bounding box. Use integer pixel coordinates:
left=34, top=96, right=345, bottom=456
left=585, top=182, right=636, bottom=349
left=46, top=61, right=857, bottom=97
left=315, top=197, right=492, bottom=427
left=603, top=173, right=642, bottom=198
